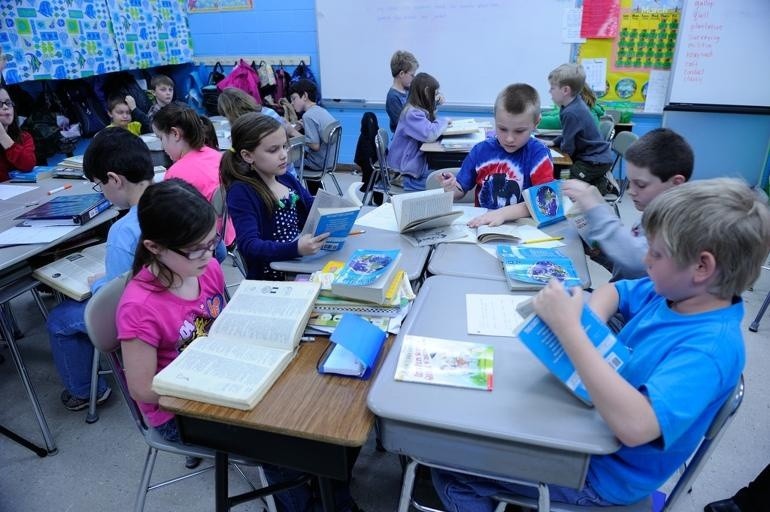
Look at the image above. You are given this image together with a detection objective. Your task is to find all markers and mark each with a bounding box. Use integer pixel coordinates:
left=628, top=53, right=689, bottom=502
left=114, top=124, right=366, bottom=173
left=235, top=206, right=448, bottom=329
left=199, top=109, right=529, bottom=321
left=332, top=99, right=342, bottom=102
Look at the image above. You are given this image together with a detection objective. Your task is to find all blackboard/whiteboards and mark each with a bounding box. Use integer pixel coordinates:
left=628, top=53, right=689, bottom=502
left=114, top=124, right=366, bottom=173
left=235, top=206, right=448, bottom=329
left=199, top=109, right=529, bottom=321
left=663, top=0, right=770, bottom=115
left=315, top=0, right=688, bottom=116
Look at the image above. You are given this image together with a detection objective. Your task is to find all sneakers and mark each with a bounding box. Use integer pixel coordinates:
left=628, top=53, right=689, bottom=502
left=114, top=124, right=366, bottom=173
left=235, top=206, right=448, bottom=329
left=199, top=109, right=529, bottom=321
left=606, top=172, right=623, bottom=195
left=61, top=387, right=112, bottom=411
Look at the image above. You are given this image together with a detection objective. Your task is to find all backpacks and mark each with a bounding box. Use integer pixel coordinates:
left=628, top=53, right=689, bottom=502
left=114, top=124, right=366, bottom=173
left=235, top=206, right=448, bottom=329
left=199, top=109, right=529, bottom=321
left=200, top=59, right=319, bottom=116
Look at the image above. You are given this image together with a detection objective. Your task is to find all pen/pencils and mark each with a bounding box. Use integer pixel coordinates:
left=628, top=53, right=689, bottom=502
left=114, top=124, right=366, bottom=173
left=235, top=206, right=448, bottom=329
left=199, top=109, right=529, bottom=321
left=300, top=336, right=315, bottom=342
left=48, top=184, right=72, bottom=195
left=9, top=180, right=36, bottom=183
left=348, top=230, right=366, bottom=235
left=520, top=236, right=565, bottom=244
left=442, top=172, right=463, bottom=192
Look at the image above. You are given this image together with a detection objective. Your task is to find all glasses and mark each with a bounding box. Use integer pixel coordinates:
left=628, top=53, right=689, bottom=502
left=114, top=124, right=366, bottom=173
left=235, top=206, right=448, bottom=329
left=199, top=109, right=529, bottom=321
left=166, top=232, right=223, bottom=261
left=0, top=99, right=14, bottom=108
left=92, top=178, right=106, bottom=192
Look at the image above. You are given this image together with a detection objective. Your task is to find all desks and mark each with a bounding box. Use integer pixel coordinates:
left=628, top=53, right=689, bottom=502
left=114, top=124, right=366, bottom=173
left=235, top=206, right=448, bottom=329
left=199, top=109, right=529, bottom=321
left=157, top=278, right=417, bottom=510
left=1, top=111, right=341, bottom=457
left=426, top=210, right=591, bottom=290
left=363, top=274, right=622, bottom=512
left=270, top=206, right=432, bottom=289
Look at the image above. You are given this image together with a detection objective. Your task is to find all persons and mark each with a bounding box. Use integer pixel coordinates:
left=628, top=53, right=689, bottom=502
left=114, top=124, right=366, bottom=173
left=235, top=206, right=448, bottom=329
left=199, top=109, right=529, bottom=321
left=432, top=178, right=770, bottom=509
left=557, top=129, right=694, bottom=276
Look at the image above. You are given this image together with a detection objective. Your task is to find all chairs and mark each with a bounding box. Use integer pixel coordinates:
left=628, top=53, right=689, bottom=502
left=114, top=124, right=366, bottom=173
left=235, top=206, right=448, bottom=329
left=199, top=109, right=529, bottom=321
left=494, top=377, right=745, bottom=512
left=353, top=108, right=640, bottom=222
left=86, top=270, right=261, bottom=510
